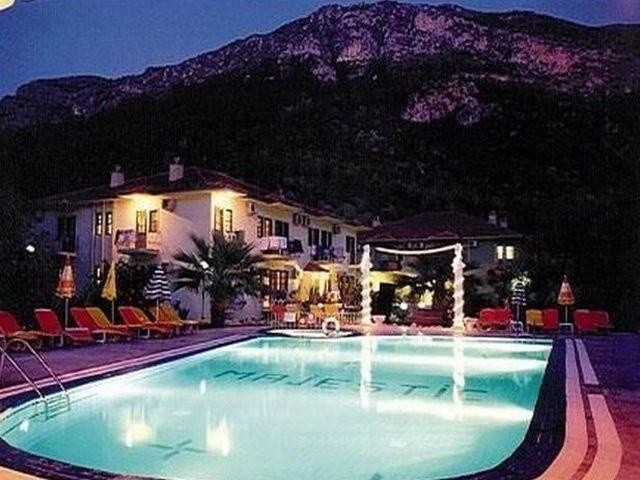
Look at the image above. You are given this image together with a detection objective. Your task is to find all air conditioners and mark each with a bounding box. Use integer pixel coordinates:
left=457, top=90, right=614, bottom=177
left=161, top=198, right=175, bottom=209
left=244, top=203, right=344, bottom=235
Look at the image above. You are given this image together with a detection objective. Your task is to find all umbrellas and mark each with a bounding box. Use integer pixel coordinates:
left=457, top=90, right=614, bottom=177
left=557, top=275, right=576, bottom=323
left=53, top=253, right=76, bottom=329
left=98, top=260, right=117, bottom=322
left=510, top=283, right=528, bottom=339
left=145, top=261, right=172, bottom=322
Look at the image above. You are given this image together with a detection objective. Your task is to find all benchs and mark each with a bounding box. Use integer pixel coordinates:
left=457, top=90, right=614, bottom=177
left=411, top=308, right=445, bottom=327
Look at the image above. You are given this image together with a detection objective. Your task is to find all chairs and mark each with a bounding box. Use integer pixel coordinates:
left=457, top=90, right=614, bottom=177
left=0, top=301, right=199, bottom=357
left=272, top=303, right=345, bottom=328
left=467, top=303, right=613, bottom=339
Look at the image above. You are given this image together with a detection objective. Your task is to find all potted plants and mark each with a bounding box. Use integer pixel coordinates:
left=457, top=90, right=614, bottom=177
left=166, top=231, right=267, bottom=330
